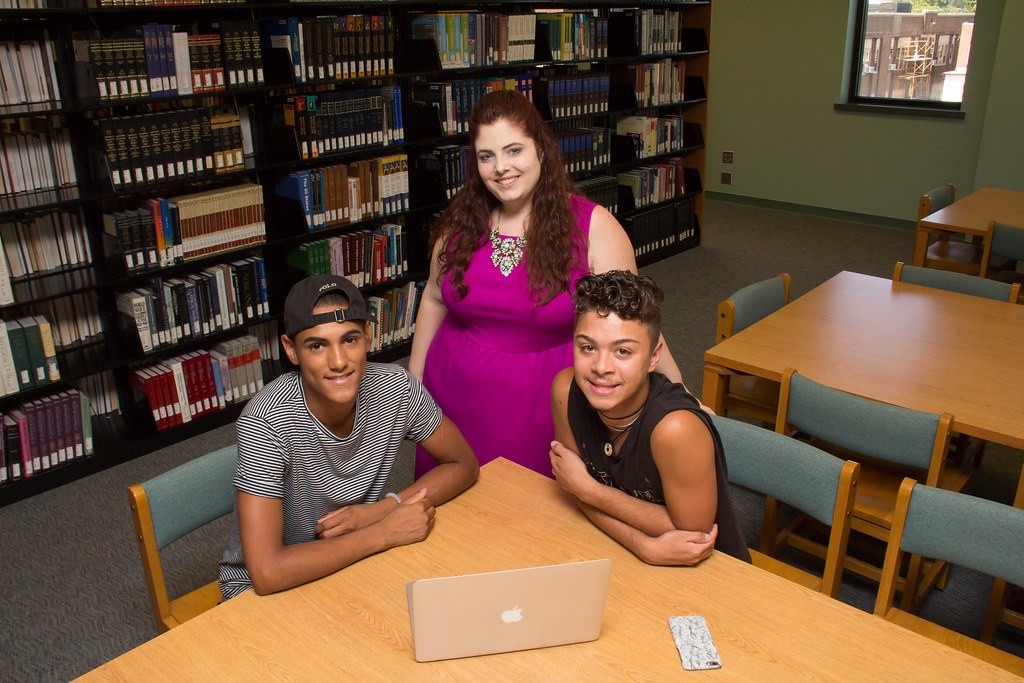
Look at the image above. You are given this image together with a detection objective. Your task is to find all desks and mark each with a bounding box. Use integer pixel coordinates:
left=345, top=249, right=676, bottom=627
left=69, top=456, right=1023, bottom=683
left=700, top=269, right=1024, bottom=505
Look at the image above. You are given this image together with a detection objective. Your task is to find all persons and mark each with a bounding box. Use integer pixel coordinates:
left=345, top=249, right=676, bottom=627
left=409, top=89, right=718, bottom=483
left=217, top=274, right=481, bottom=604
left=549, top=270, right=750, bottom=567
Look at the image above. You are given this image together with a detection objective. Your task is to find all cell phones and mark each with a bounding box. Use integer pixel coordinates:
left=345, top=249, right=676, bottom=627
left=668, top=616, right=721, bottom=670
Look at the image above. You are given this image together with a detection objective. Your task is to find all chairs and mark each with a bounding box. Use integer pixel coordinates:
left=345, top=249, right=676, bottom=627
left=979, top=220, right=1024, bottom=278
left=872, top=478, right=1023, bottom=677
left=911, top=184, right=1017, bottom=276
left=892, top=262, right=1021, bottom=469
left=715, top=272, right=792, bottom=424
left=758, top=367, right=972, bottom=616
left=706, top=415, right=860, bottom=600
left=980, top=460, right=1024, bottom=648
left=128, top=443, right=237, bottom=635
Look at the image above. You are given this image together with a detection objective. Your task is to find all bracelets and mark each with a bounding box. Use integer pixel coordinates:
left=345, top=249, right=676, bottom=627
left=385, top=493, right=401, bottom=504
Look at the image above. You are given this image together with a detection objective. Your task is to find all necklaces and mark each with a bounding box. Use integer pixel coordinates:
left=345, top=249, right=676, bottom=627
left=596, top=396, right=649, bottom=456
left=488, top=203, right=529, bottom=276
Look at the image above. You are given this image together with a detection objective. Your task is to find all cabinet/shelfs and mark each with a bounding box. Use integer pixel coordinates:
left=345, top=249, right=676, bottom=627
left=1, top=1, right=713, bottom=508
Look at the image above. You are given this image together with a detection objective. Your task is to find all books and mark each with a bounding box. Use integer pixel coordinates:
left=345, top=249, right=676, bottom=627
left=410, top=8, right=608, bottom=69
left=0, top=291, right=103, bottom=398
left=0, top=0, right=64, bottom=105
left=0, top=130, right=76, bottom=195
left=99, top=157, right=429, bottom=432
left=423, top=73, right=609, bottom=137
left=87, top=2, right=407, bottom=187
left=624, top=205, right=697, bottom=261
left=612, top=7, right=683, bottom=55
left=433, top=118, right=618, bottom=219
left=0, top=207, right=94, bottom=305
left=615, top=112, right=686, bottom=207
left=0, top=369, right=121, bottom=484
left=629, top=57, right=686, bottom=107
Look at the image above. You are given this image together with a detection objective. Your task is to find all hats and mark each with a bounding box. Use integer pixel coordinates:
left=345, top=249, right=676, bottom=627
left=283, top=275, right=378, bottom=336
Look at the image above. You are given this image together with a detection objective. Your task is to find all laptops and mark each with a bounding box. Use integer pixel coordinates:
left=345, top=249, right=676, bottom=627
left=407, top=559, right=612, bottom=664
